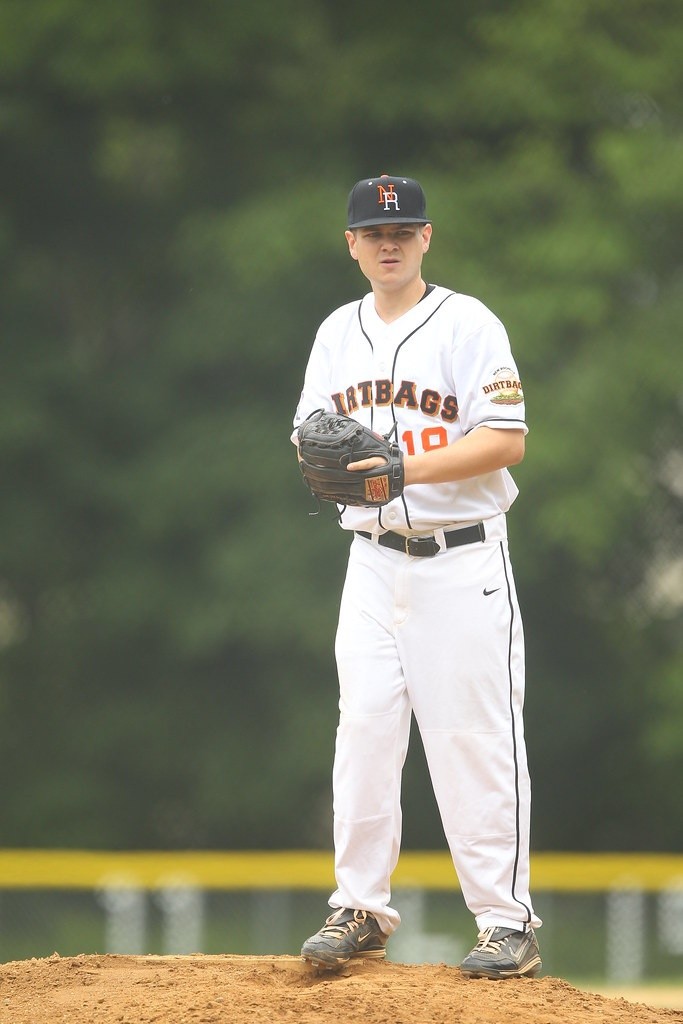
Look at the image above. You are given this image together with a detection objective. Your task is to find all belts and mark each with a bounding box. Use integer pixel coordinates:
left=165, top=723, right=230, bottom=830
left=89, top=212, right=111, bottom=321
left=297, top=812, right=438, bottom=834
left=355, top=522, right=485, bottom=556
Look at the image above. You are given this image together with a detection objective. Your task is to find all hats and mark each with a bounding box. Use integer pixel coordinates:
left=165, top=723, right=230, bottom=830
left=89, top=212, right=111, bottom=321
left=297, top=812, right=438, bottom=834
left=347, top=175, right=432, bottom=228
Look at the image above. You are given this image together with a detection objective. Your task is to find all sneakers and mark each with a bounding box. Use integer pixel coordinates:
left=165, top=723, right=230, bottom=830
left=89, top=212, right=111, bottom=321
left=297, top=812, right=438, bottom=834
left=460, top=926, right=542, bottom=980
left=300, top=904, right=389, bottom=969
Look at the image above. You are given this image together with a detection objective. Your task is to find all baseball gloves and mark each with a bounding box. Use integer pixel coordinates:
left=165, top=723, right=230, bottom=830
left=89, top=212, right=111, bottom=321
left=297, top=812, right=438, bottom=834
left=296, top=411, right=404, bottom=510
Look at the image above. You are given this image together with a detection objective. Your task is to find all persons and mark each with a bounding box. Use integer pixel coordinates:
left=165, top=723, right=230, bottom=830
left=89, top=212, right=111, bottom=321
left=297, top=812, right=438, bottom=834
left=287, top=174, right=543, bottom=981
